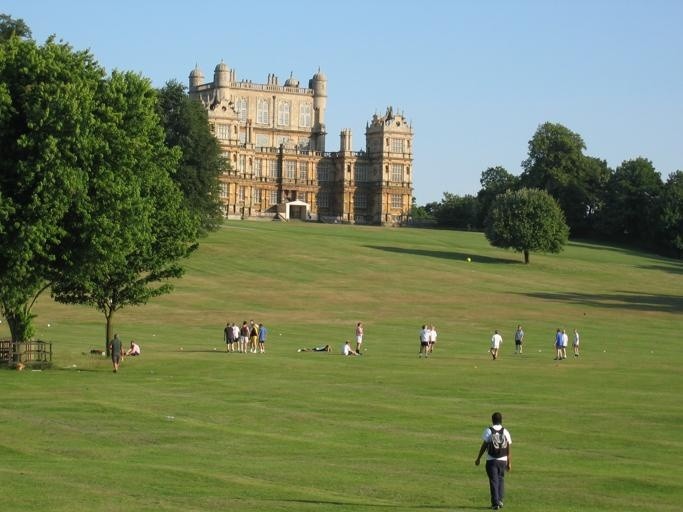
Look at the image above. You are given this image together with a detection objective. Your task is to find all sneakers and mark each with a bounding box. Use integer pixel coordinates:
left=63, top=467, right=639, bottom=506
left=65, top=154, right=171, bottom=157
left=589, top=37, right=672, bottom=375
left=493, top=355, right=497, bottom=360
left=554, top=356, right=566, bottom=360
left=227, top=348, right=265, bottom=354
left=355, top=352, right=362, bottom=356
left=575, top=353, right=579, bottom=357
left=418, top=350, right=432, bottom=360
left=113, top=369, right=116, bottom=372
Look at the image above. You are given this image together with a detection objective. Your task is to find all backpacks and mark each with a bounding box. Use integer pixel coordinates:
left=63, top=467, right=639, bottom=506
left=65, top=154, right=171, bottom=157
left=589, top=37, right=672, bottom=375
left=487, top=426, right=510, bottom=458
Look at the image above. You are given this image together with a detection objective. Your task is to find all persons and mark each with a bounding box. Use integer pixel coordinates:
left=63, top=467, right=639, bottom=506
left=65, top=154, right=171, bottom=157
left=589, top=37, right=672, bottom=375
left=223, top=320, right=266, bottom=353
left=552, top=327, right=562, bottom=360
left=514, top=325, right=524, bottom=353
left=418, top=324, right=429, bottom=357
left=311, top=344, right=332, bottom=352
left=427, top=326, right=437, bottom=352
left=342, top=339, right=360, bottom=356
left=107, top=333, right=123, bottom=373
left=473, top=412, right=512, bottom=510
left=572, top=328, right=579, bottom=356
left=354, top=322, right=363, bottom=354
left=561, top=329, right=568, bottom=359
left=124, top=340, right=140, bottom=355
left=489, top=329, right=502, bottom=360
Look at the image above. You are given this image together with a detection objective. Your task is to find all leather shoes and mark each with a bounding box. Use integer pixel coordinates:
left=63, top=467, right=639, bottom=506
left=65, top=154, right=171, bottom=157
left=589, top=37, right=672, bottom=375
left=488, top=501, right=503, bottom=510
